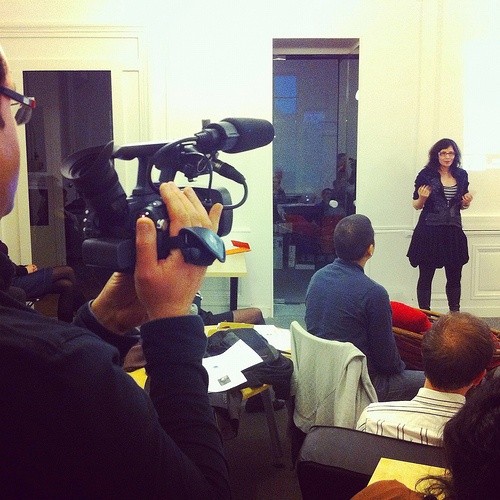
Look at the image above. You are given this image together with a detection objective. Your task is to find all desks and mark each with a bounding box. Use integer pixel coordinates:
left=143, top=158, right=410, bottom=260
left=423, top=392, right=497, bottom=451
left=204, top=254, right=247, bottom=311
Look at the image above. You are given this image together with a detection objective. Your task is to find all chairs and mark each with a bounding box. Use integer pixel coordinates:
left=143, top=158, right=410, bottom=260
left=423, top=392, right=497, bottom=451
left=290, top=321, right=378, bottom=432
left=297, top=424, right=451, bottom=500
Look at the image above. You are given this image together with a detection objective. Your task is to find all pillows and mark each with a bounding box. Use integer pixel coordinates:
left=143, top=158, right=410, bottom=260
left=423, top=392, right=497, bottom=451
left=390, top=301, right=431, bottom=333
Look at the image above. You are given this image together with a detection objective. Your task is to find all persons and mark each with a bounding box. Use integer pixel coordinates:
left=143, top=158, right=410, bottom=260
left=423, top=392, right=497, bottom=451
left=0, top=52, right=230, bottom=500
left=406, top=137, right=474, bottom=317
left=0, top=241, right=78, bottom=322
left=303, top=213, right=426, bottom=403
left=271, top=153, right=358, bottom=274
left=350, top=311, right=500, bottom=500
left=193, top=292, right=264, bottom=329
left=30, top=174, right=86, bottom=257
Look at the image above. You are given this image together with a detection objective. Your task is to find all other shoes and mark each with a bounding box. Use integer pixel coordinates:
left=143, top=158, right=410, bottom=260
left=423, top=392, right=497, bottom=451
left=246, top=399, right=285, bottom=412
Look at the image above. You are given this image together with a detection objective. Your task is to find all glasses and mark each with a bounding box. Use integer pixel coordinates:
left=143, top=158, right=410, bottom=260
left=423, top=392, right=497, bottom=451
left=438, top=150, right=457, bottom=158
left=273, top=180, right=281, bottom=184
left=0, top=84, right=37, bottom=125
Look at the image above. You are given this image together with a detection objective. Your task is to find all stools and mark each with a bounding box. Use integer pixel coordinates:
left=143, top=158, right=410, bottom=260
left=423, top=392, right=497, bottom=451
left=127, top=367, right=285, bottom=470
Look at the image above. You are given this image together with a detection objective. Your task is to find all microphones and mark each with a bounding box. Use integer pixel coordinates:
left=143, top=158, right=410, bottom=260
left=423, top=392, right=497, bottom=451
left=194, top=117, right=275, bottom=153
